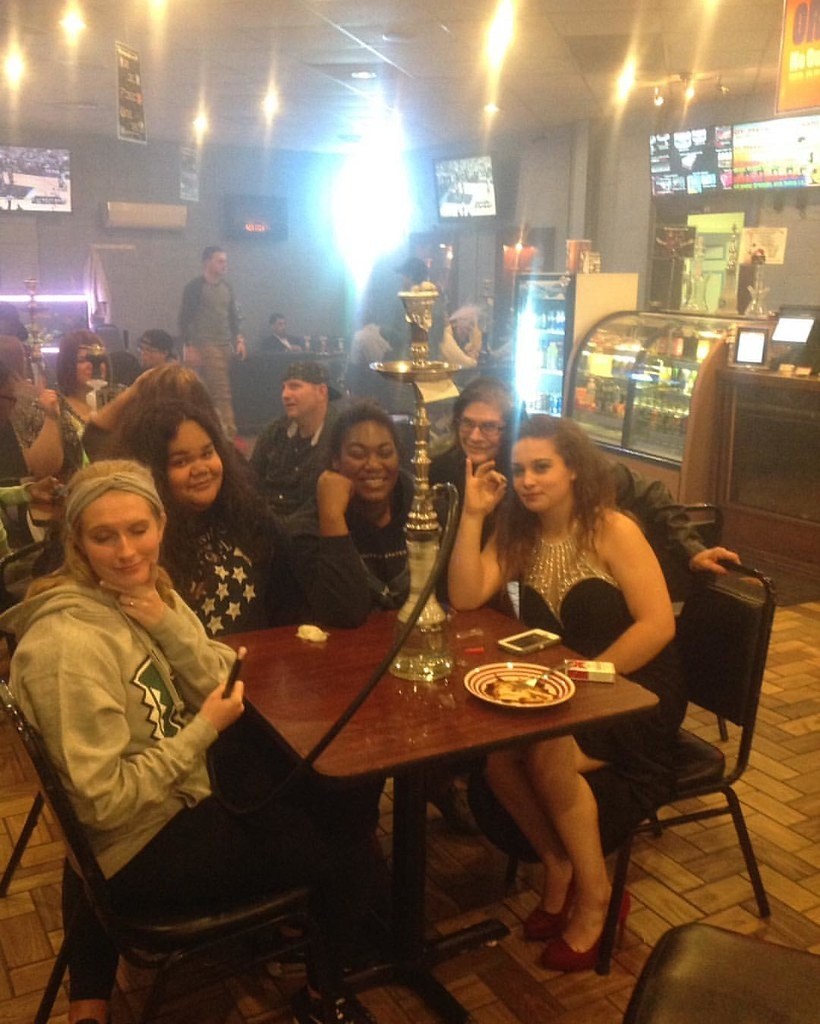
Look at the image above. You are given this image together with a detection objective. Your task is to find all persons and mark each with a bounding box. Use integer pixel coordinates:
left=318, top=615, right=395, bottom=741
left=447, top=413, right=688, bottom=972
left=350, top=257, right=492, bottom=364
left=139, top=328, right=173, bottom=368
left=0, top=456, right=378, bottom=1024
left=0, top=300, right=128, bottom=481
left=81, top=364, right=253, bottom=479
left=272, top=400, right=479, bottom=839
left=266, top=314, right=303, bottom=350
left=425, top=376, right=740, bottom=602
left=177, top=245, right=249, bottom=438
left=249, top=359, right=342, bottom=479
left=0, top=395, right=310, bottom=1024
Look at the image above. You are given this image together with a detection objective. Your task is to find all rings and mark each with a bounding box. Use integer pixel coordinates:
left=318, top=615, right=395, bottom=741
left=130, top=598, right=136, bottom=606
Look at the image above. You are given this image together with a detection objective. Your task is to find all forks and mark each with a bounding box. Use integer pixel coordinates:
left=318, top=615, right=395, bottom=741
left=513, top=661, right=572, bottom=690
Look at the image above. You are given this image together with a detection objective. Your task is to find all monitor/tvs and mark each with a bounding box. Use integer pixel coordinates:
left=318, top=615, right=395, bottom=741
left=0, top=144, right=73, bottom=213
left=433, top=153, right=498, bottom=222
left=771, top=318, right=815, bottom=344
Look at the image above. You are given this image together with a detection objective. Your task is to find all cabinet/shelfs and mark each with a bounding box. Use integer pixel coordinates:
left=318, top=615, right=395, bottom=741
left=557, top=309, right=730, bottom=522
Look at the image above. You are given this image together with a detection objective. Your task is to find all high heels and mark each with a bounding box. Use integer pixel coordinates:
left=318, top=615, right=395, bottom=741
left=547, top=883, right=631, bottom=971
left=523, top=870, right=575, bottom=939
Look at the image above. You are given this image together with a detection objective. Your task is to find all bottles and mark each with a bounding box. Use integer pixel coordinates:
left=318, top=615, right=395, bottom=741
left=522, top=310, right=565, bottom=371
left=584, top=378, right=626, bottom=419
left=633, top=398, right=690, bottom=435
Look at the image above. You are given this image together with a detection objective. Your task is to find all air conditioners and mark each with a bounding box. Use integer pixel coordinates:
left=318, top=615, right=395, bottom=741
left=102, top=200, right=189, bottom=231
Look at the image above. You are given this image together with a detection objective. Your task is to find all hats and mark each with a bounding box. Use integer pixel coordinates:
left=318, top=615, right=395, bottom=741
left=280, top=361, right=342, bottom=402
left=141, top=329, right=177, bottom=355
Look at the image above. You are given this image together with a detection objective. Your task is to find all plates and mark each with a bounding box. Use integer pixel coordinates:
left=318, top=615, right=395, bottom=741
left=464, top=662, right=576, bottom=708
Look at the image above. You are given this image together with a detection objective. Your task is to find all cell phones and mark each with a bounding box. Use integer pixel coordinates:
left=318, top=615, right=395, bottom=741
left=497, top=629, right=562, bottom=656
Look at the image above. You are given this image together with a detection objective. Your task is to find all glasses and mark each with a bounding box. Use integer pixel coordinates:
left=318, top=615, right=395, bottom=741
left=457, top=418, right=506, bottom=436
left=136, top=348, right=158, bottom=354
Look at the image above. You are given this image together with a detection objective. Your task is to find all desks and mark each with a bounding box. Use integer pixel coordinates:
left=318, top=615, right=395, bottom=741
left=214, top=600, right=662, bottom=1024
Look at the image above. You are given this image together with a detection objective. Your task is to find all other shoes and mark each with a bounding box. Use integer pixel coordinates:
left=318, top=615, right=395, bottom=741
left=425, top=776, right=483, bottom=835
left=290, top=986, right=377, bottom=1024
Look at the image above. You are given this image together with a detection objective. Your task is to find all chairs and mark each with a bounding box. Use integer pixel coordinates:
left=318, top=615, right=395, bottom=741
left=508, top=504, right=819, bottom=1024
left=0, top=538, right=54, bottom=657
left=1, top=679, right=316, bottom=1024
